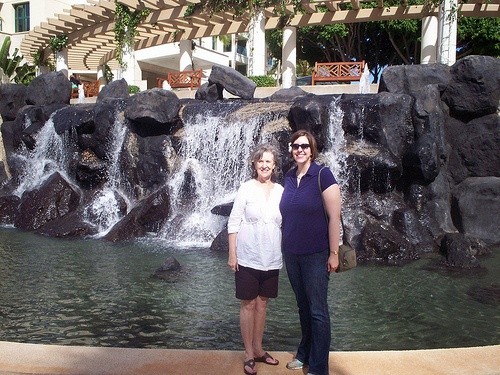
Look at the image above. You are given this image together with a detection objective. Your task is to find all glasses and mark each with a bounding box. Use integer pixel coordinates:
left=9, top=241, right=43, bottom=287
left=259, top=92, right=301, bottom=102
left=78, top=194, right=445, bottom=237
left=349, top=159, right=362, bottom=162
left=290, top=144, right=311, bottom=149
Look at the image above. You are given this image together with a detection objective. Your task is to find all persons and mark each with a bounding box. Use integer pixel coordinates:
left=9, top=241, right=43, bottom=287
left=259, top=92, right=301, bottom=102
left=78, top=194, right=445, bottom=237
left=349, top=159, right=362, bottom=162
left=227, top=144, right=284, bottom=375
left=279, top=129, right=340, bottom=375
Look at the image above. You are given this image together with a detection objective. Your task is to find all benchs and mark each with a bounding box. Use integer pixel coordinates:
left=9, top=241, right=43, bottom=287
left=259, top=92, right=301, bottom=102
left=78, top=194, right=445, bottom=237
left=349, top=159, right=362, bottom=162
left=312, top=60, right=365, bottom=86
left=156, top=68, right=202, bottom=91
left=71, top=80, right=101, bottom=98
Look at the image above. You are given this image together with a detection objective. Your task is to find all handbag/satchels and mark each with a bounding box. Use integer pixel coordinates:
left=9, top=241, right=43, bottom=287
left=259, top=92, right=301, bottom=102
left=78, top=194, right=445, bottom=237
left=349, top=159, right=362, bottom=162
left=335, top=242, right=357, bottom=273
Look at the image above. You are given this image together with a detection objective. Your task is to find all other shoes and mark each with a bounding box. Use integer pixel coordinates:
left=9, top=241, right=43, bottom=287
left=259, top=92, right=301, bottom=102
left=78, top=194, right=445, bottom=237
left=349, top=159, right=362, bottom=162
left=286, top=359, right=308, bottom=370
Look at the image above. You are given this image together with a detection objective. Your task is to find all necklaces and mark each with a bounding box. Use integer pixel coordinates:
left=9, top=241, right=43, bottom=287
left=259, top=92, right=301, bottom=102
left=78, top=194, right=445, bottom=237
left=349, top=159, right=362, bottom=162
left=297, top=176, right=302, bottom=180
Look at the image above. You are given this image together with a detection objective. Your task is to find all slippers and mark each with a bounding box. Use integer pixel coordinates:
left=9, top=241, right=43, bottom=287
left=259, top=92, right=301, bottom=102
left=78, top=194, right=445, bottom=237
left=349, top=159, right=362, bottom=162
left=254, top=352, right=279, bottom=366
left=244, top=358, right=258, bottom=375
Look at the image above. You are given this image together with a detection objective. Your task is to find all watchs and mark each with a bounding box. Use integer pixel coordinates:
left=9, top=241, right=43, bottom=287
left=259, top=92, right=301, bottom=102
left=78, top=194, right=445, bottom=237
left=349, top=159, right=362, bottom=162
left=329, top=251, right=339, bottom=255
